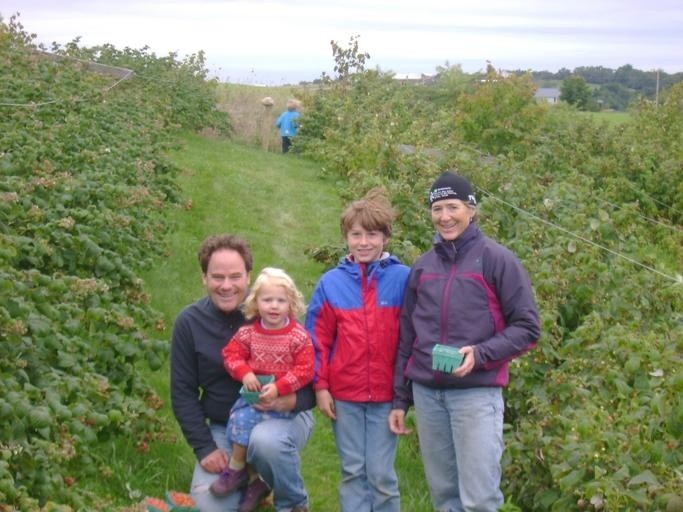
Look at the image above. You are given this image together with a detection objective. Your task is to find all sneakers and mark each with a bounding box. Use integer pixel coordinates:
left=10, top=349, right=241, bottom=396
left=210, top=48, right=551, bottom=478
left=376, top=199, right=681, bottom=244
left=211, top=465, right=249, bottom=494
left=239, top=477, right=269, bottom=512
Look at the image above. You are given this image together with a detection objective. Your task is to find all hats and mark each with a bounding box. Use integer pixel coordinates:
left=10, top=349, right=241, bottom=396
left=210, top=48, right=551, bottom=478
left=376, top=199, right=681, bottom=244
left=430, top=171, right=476, bottom=206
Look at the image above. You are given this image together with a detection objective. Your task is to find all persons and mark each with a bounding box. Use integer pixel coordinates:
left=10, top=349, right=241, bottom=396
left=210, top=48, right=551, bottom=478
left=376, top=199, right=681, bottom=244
left=210, top=267, right=316, bottom=511
left=304, top=198, right=413, bottom=511
left=170, top=232, right=318, bottom=512
left=273, top=99, right=302, bottom=156
left=388, top=170, right=540, bottom=511
